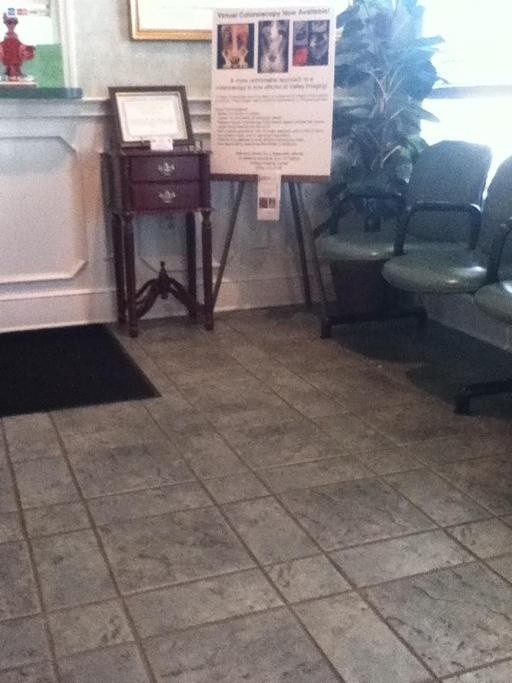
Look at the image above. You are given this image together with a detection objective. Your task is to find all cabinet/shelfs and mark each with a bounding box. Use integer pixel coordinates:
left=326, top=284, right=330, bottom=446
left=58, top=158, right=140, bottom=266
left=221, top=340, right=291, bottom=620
left=99, top=145, right=215, bottom=339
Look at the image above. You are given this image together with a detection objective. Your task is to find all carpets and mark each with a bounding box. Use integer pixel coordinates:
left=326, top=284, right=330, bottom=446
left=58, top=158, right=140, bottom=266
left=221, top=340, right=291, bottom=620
left=0, top=323, right=160, bottom=419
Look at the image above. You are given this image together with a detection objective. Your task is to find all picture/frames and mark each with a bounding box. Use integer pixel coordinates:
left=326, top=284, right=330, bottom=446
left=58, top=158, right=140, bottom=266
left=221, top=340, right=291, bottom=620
left=107, top=86, right=196, bottom=147
left=129, top=0, right=212, bottom=41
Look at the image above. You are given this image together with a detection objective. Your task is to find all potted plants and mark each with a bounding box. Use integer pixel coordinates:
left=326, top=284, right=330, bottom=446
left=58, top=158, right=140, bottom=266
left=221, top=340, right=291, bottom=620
left=314, top=0, right=451, bottom=321
